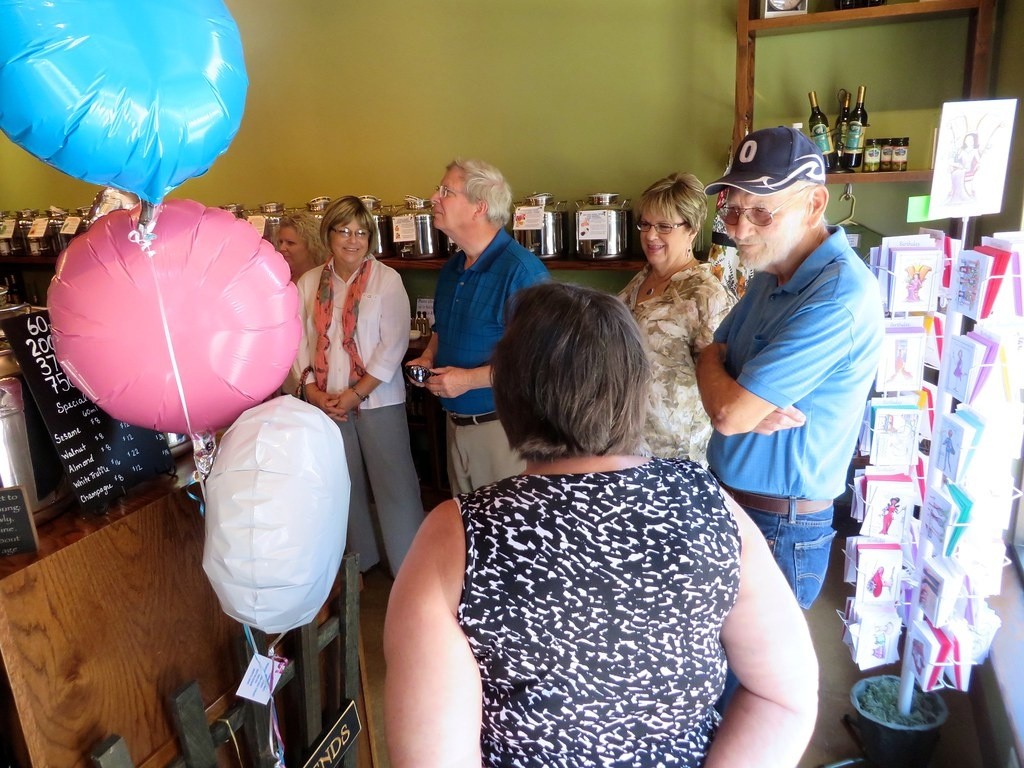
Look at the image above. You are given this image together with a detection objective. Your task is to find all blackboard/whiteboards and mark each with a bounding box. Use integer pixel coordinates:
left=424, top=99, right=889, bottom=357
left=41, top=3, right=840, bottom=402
left=0, top=486, right=40, bottom=557
left=0, top=308, right=176, bottom=514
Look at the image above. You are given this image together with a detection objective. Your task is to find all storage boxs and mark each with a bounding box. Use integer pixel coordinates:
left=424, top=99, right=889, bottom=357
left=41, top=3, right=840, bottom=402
left=759, top=0, right=809, bottom=19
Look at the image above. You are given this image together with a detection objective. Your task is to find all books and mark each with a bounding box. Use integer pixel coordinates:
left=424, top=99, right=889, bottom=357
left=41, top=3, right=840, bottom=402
left=839, top=227, right=1024, bottom=695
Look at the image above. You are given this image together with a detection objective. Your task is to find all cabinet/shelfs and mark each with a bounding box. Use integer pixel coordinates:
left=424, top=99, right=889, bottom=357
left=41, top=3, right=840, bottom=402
left=734, top=0, right=994, bottom=185
left=381, top=252, right=646, bottom=487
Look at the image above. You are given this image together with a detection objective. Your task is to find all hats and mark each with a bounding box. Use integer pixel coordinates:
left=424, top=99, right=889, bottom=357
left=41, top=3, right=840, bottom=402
left=704, top=127, right=825, bottom=196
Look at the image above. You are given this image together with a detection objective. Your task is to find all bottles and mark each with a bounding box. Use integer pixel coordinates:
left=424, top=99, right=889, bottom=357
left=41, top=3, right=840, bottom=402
left=574, top=192, right=633, bottom=260
left=841, top=85, right=869, bottom=169
left=808, top=89, right=835, bottom=169
left=218, top=195, right=463, bottom=260
left=833, top=92, right=851, bottom=168
left=411, top=311, right=431, bottom=337
left=511, top=192, right=568, bottom=259
left=0, top=205, right=94, bottom=258
left=4, top=274, right=39, bottom=306
left=792, top=122, right=803, bottom=131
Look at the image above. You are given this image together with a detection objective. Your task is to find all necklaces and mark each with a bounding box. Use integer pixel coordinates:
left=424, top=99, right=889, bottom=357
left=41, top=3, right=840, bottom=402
left=647, top=258, right=694, bottom=296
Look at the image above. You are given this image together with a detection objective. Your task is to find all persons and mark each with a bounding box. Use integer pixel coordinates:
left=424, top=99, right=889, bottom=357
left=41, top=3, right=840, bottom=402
left=272, top=213, right=329, bottom=397
left=383, top=280, right=819, bottom=768
left=292, top=196, right=424, bottom=581
left=615, top=171, right=739, bottom=471
left=405, top=159, right=553, bottom=497
left=695, top=125, right=885, bottom=720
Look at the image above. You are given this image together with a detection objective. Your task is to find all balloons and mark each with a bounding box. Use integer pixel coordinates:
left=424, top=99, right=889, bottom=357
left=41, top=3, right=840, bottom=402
left=201, top=395, right=352, bottom=635
left=46, top=197, right=302, bottom=438
left=0, top=0, right=249, bottom=205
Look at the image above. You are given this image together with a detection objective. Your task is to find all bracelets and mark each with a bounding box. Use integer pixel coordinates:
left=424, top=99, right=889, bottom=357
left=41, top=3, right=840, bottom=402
left=353, top=386, right=369, bottom=402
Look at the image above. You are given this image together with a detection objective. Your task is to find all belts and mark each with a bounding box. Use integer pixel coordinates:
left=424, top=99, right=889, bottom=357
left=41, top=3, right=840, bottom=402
left=450, top=411, right=499, bottom=426
left=710, top=468, right=833, bottom=514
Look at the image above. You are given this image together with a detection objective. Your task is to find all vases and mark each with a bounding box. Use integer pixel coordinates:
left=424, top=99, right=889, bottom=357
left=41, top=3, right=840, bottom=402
left=850, top=674, right=948, bottom=761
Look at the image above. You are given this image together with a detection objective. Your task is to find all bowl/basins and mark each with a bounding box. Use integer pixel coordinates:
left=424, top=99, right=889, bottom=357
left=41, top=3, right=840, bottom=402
left=409, top=330, right=421, bottom=340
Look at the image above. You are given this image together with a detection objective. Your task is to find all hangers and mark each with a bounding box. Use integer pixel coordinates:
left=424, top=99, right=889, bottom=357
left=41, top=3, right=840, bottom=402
left=836, top=194, right=859, bottom=227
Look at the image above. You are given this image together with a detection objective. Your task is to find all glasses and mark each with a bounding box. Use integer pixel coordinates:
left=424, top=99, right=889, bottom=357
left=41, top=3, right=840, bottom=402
left=433, top=186, right=481, bottom=200
left=330, top=227, right=369, bottom=238
left=717, top=185, right=816, bottom=226
left=637, top=217, right=688, bottom=234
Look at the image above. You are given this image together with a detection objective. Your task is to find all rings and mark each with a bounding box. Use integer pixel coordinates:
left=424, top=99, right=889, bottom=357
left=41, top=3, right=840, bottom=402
left=438, top=392, right=441, bottom=398
